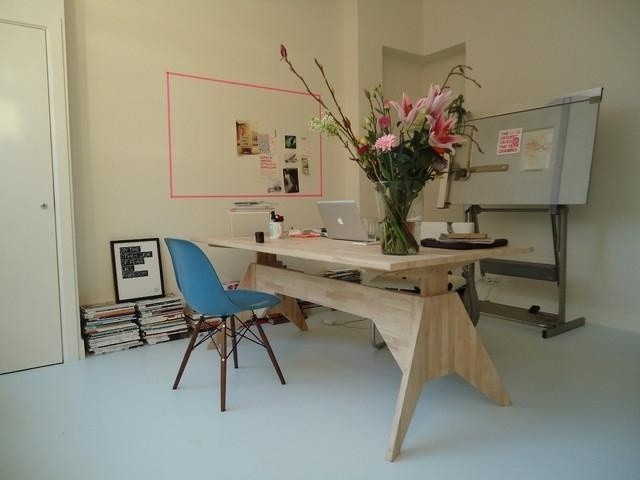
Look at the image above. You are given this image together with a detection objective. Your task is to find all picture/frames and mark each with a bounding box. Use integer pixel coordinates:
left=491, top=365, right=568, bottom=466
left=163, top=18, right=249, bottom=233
left=109, top=238, right=165, bottom=304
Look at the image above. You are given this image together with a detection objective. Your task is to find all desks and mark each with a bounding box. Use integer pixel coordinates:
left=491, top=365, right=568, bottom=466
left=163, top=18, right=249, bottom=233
left=208, top=234, right=535, bottom=467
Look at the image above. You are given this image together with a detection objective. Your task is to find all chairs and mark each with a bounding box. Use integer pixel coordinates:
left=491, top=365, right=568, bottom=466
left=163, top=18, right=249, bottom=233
left=164, top=238, right=287, bottom=412
left=370, top=220, right=480, bottom=349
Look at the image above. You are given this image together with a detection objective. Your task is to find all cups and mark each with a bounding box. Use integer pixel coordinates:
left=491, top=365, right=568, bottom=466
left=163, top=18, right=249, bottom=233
left=451, top=222, right=475, bottom=233
left=254, top=232, right=264, bottom=244
left=268, top=221, right=283, bottom=239
left=363, top=217, right=377, bottom=242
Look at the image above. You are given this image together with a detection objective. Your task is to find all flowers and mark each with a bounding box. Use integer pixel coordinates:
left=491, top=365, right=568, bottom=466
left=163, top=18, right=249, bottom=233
left=278, top=44, right=486, bottom=253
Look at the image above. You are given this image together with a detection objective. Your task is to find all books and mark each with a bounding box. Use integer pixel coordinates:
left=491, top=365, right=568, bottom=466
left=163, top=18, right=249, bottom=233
left=80, top=269, right=363, bottom=356
left=433, top=232, right=495, bottom=244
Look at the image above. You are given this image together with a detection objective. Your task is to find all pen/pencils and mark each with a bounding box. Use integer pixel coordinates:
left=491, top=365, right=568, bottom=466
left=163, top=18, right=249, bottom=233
left=271, top=210, right=284, bottom=221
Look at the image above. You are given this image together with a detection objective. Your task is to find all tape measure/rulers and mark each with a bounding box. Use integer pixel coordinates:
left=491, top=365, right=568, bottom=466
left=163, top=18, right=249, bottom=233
left=470, top=164, right=509, bottom=173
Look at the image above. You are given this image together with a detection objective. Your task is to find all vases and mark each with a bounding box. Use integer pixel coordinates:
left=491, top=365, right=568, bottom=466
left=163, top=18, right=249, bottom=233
left=373, top=181, right=427, bottom=256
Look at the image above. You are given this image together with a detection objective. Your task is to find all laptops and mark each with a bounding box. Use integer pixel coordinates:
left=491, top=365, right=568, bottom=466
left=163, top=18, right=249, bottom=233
left=316, top=200, right=376, bottom=242
